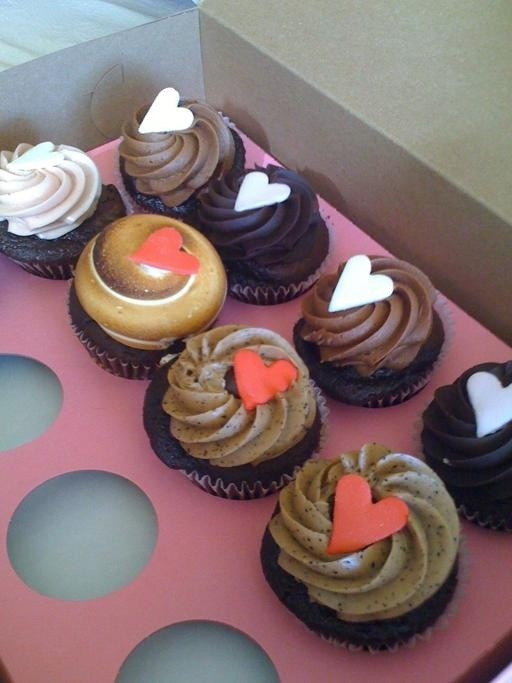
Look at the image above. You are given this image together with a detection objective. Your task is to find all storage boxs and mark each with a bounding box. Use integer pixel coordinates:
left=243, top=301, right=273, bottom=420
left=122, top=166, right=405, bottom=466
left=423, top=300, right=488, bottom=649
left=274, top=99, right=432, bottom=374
left=0, top=0, right=510, bottom=682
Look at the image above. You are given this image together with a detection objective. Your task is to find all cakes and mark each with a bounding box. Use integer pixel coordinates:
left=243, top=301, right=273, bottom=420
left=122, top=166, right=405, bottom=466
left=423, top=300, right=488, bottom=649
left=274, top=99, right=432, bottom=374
left=191, top=162, right=336, bottom=306
left=419, top=361, right=512, bottom=533
left=118, top=86, right=246, bottom=236
left=259, top=440, right=471, bottom=653
left=142, top=321, right=330, bottom=501
left=0, top=139, right=127, bottom=281
left=293, top=253, right=456, bottom=410
left=67, top=214, right=229, bottom=381
